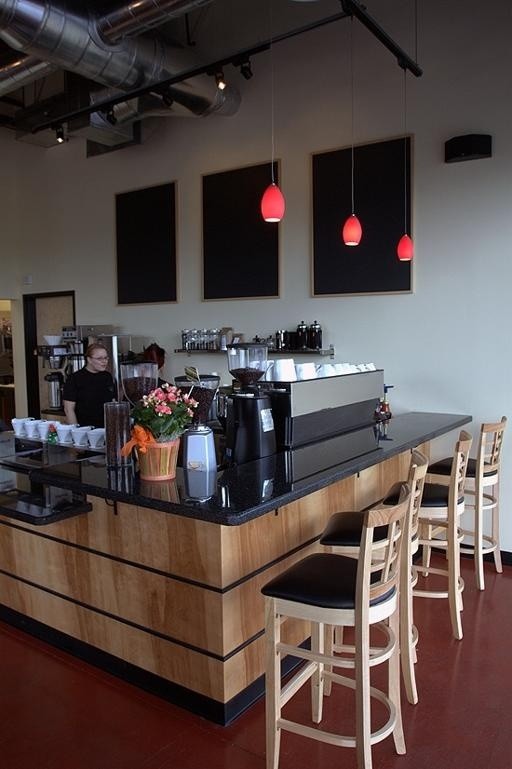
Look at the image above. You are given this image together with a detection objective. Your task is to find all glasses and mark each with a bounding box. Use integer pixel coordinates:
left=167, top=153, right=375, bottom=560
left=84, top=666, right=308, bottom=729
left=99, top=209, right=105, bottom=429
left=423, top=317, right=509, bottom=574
left=92, top=356, right=109, bottom=361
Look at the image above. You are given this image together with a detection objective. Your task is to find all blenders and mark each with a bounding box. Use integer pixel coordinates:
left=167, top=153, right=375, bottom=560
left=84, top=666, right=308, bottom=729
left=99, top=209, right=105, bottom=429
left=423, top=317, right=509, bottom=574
left=175, top=375, right=221, bottom=502
left=227, top=343, right=276, bottom=459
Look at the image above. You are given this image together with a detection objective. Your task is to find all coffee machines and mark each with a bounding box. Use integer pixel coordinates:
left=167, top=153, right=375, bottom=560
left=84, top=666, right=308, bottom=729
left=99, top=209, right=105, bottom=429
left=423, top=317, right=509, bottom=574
left=37, top=324, right=119, bottom=416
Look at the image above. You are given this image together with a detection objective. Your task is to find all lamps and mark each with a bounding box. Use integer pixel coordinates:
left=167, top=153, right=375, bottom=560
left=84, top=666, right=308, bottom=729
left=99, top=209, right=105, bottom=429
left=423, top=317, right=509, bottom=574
left=260, top=43, right=287, bottom=224
left=397, top=64, right=415, bottom=261
left=342, top=9, right=363, bottom=247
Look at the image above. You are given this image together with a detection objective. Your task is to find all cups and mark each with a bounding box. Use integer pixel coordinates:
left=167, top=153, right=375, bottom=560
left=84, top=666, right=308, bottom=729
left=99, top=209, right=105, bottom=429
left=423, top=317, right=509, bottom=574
left=15, top=501, right=28, bottom=515
left=29, top=505, right=41, bottom=517
left=56, top=424, right=73, bottom=442
left=274, top=358, right=296, bottom=382
left=322, top=362, right=377, bottom=377
left=38, top=421, right=56, bottom=440
left=87, top=455, right=105, bottom=464
left=10, top=417, right=24, bottom=434
left=72, top=423, right=89, bottom=446
left=297, top=362, right=318, bottom=379
left=87, top=428, right=105, bottom=448
left=24, top=421, right=39, bottom=436
left=41, top=508, right=51, bottom=516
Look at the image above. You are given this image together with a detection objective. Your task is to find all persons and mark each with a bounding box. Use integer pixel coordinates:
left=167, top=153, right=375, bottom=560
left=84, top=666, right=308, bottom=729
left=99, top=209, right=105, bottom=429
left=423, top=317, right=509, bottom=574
left=122, top=341, right=173, bottom=405
left=63, top=344, right=118, bottom=429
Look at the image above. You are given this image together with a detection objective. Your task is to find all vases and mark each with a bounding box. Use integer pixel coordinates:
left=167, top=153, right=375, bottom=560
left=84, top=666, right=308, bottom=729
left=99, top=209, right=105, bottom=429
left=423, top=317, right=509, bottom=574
left=136, top=441, right=179, bottom=482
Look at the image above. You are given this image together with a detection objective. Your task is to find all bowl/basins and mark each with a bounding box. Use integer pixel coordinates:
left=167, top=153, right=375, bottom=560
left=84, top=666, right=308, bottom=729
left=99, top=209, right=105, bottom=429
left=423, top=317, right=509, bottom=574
left=43, top=335, right=62, bottom=346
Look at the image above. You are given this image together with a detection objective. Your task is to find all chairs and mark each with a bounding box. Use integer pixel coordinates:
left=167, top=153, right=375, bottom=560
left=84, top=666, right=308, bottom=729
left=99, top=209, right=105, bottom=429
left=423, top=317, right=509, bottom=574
left=429, top=415, right=506, bottom=591
left=261, top=482, right=411, bottom=769
left=320, top=445, right=427, bottom=706
left=384, top=431, right=479, bottom=640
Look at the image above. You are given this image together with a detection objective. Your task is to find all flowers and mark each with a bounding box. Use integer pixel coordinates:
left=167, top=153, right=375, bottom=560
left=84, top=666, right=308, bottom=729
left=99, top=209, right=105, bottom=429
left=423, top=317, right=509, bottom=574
left=128, top=382, right=198, bottom=442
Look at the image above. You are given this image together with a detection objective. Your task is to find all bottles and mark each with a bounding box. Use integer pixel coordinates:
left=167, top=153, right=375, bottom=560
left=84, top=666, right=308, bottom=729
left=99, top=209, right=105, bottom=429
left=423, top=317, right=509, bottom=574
left=310, top=320, right=322, bottom=349
left=210, top=328, right=218, bottom=349
left=182, top=329, right=190, bottom=350
left=200, top=329, right=208, bottom=349
left=191, top=329, right=199, bottom=350
left=296, top=321, right=308, bottom=348
